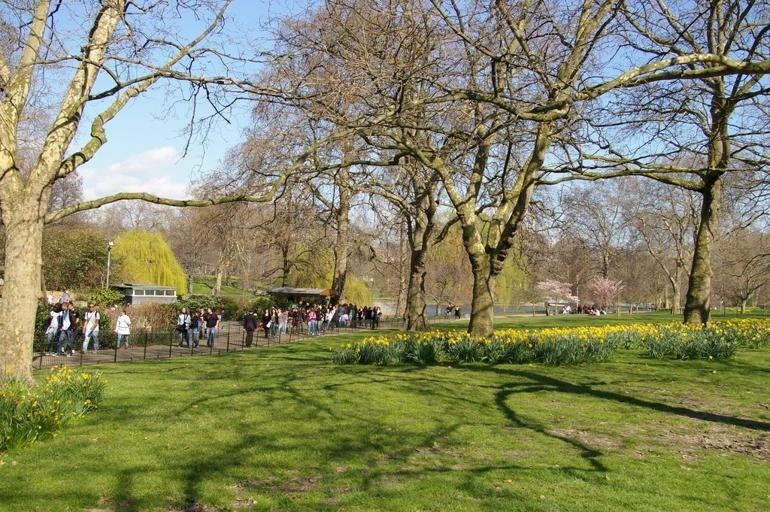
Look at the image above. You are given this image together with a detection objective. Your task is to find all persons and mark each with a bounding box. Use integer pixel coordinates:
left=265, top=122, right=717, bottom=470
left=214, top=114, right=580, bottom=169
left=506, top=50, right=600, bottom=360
left=447, top=304, right=455, bottom=316
left=455, top=307, right=461, bottom=319
left=578, top=304, right=596, bottom=314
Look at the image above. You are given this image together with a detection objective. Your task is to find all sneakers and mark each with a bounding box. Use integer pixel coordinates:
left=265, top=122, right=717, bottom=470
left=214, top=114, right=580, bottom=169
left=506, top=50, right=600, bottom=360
left=45, top=347, right=75, bottom=357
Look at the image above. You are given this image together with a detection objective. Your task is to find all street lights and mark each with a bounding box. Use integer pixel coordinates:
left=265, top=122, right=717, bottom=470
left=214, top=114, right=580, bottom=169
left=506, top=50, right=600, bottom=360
left=106, top=241, right=114, bottom=291
